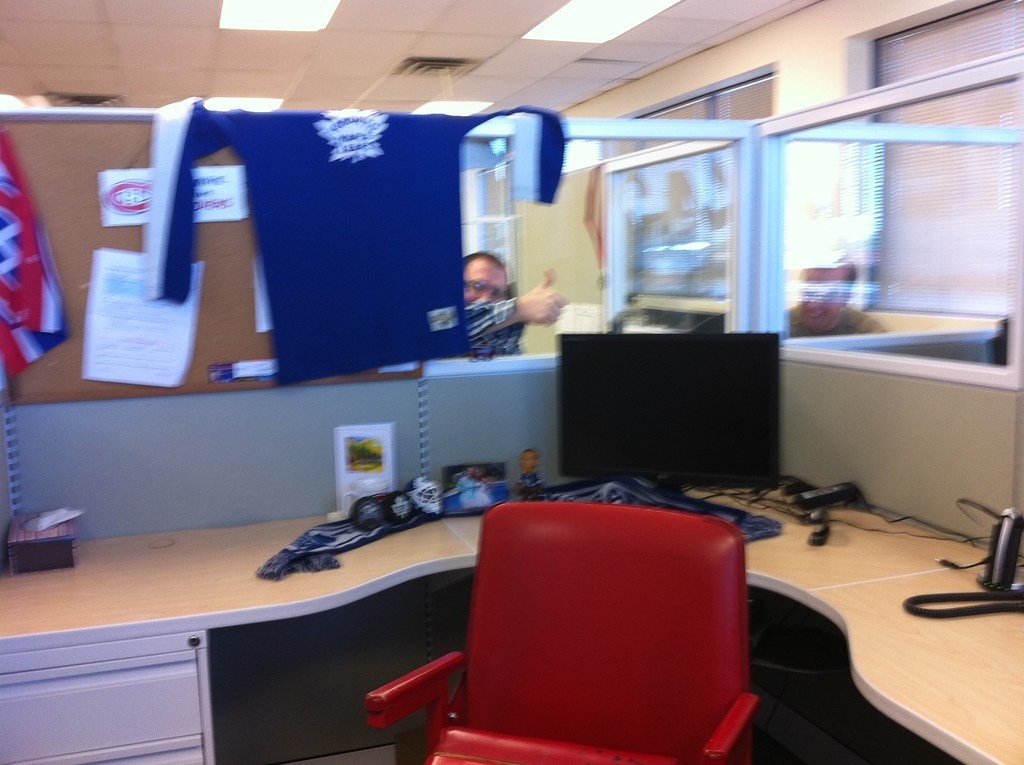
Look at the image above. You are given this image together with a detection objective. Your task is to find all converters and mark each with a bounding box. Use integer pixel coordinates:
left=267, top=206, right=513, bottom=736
left=797, top=482, right=859, bottom=510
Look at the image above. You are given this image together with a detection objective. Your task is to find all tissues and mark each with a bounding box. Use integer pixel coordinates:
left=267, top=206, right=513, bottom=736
left=7, top=506, right=85, bottom=577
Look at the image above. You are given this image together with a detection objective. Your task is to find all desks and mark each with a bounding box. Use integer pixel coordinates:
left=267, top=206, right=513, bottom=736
left=0, top=490, right=1024, bottom=765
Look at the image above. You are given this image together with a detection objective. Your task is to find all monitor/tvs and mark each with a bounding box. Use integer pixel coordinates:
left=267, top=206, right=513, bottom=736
left=557, top=334, right=781, bottom=491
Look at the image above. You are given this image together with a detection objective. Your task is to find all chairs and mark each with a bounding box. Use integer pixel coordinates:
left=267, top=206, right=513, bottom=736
left=363, top=501, right=761, bottom=765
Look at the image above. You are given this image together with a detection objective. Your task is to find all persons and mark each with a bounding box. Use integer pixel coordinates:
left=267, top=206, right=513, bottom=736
left=456, top=468, right=493, bottom=509
left=511, top=449, right=544, bottom=501
left=463, top=249, right=567, bottom=358
left=786, top=250, right=886, bottom=338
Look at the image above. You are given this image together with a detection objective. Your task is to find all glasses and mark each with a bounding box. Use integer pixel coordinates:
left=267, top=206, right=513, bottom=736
left=466, top=279, right=505, bottom=301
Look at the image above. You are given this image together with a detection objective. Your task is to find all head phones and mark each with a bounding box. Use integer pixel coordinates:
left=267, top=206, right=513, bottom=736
left=350, top=491, right=420, bottom=533
